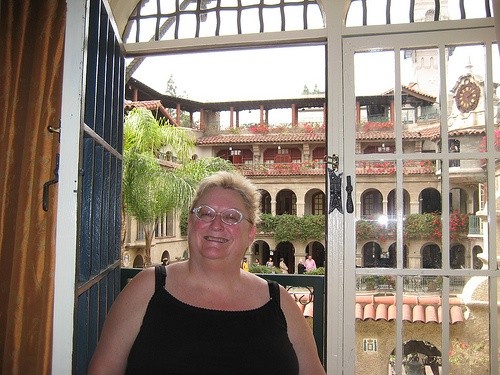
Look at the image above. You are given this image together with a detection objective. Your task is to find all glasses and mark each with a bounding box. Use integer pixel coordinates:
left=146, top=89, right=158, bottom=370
left=193, top=205, right=251, bottom=226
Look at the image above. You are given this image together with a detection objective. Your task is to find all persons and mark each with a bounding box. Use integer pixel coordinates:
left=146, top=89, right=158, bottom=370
left=86, top=170, right=327, bottom=375
left=161, top=257, right=169, bottom=266
left=240, top=254, right=316, bottom=274
